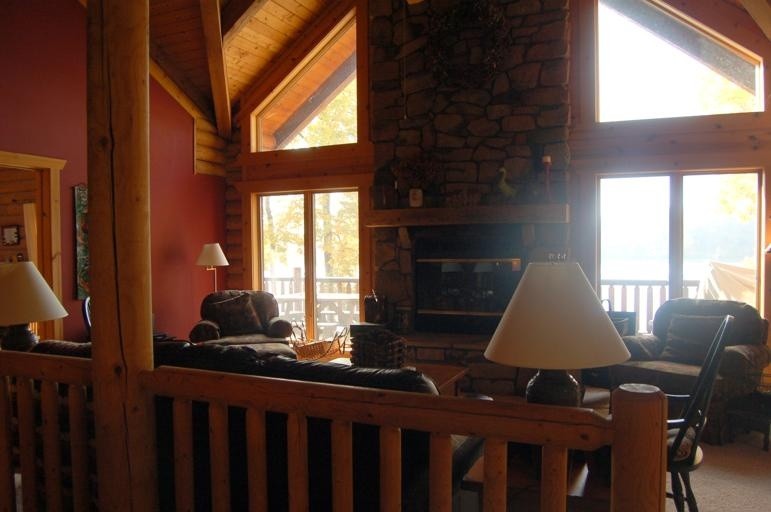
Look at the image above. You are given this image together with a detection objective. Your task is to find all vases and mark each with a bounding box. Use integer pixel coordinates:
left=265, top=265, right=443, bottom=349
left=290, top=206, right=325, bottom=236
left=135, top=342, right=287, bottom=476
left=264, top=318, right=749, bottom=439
left=408, top=189, right=424, bottom=208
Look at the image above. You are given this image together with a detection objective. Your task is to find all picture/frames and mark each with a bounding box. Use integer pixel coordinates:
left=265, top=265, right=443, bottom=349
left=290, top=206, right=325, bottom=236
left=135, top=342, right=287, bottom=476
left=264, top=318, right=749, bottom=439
left=2, top=224, right=21, bottom=246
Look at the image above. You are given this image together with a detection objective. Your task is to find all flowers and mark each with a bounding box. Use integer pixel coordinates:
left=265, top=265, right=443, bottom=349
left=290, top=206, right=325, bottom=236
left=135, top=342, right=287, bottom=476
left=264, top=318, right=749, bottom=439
left=392, top=149, right=438, bottom=190
left=426, top=1, right=511, bottom=85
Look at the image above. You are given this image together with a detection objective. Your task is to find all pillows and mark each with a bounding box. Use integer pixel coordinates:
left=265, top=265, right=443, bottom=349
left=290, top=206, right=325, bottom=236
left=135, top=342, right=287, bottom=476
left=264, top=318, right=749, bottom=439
left=660, top=313, right=725, bottom=370
left=211, top=293, right=261, bottom=335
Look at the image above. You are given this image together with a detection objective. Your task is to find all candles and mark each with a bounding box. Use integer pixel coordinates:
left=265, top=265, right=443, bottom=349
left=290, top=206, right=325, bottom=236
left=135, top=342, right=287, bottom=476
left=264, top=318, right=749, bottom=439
left=542, top=155, right=552, bottom=163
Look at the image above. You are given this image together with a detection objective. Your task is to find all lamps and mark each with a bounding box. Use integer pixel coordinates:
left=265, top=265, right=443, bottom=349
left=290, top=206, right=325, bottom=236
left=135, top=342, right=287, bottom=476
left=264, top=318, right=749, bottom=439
left=0, top=262, right=68, bottom=349
left=484, top=262, right=630, bottom=477
left=196, top=242, right=230, bottom=292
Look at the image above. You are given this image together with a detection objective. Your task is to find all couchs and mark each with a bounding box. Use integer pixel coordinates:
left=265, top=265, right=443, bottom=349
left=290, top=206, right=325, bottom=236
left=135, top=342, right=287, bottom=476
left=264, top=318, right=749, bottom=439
left=30, top=340, right=494, bottom=512
left=610, top=299, right=771, bottom=445
left=189, top=290, right=293, bottom=344
left=217, top=342, right=298, bottom=358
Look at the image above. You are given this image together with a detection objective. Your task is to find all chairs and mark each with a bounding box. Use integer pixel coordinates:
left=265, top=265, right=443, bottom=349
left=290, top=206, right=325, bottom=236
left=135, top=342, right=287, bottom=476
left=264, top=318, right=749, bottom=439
left=587, top=313, right=734, bottom=511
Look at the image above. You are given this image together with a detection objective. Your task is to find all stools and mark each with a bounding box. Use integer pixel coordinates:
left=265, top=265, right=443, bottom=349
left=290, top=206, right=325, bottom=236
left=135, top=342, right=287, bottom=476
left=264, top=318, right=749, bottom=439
left=724, top=391, right=771, bottom=451
left=460, top=454, right=612, bottom=512
